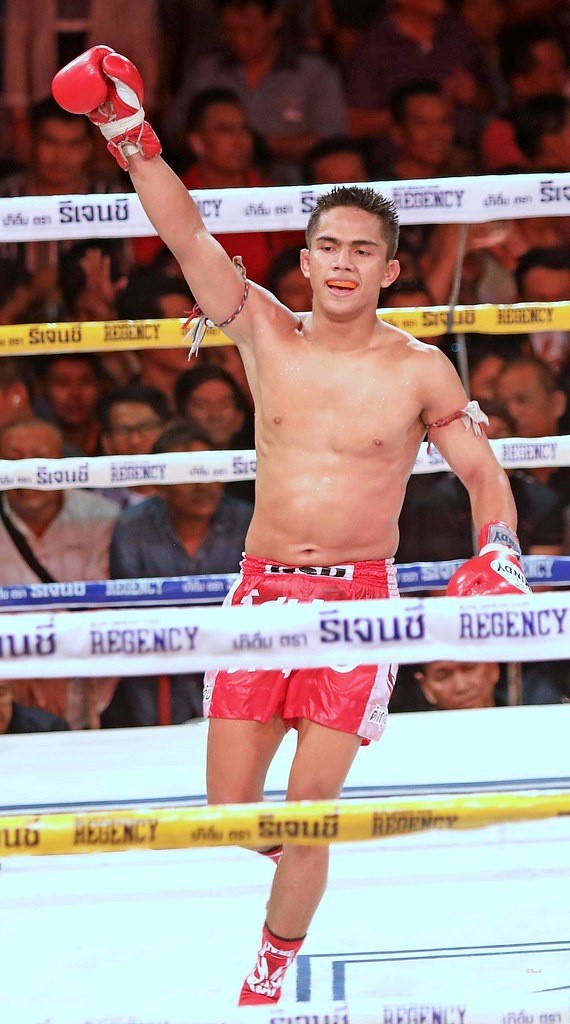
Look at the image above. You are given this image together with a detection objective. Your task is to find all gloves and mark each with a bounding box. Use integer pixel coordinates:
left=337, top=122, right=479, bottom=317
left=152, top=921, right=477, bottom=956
left=50, top=45, right=163, bottom=172
left=445, top=519, right=534, bottom=602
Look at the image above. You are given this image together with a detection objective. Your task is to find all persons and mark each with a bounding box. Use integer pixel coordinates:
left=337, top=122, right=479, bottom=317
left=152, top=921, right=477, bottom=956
left=0, top=0, right=570, bottom=735
left=50, top=44, right=533, bottom=1006
